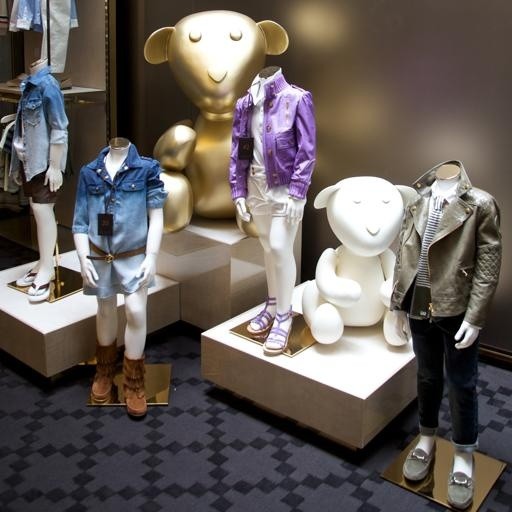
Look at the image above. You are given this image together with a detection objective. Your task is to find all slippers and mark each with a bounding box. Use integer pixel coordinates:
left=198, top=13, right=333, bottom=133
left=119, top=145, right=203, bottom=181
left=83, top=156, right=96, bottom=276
left=17, top=267, right=50, bottom=302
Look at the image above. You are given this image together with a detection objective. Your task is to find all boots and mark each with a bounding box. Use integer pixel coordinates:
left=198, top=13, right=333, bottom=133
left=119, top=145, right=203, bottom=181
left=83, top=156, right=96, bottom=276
left=92, top=342, right=147, bottom=418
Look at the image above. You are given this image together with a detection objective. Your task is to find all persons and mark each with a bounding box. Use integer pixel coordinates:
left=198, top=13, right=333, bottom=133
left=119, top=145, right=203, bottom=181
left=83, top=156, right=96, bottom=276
left=390, top=160, right=502, bottom=509
left=229, top=66, right=316, bottom=356
left=8, top=57, right=69, bottom=302
left=72, top=138, right=169, bottom=417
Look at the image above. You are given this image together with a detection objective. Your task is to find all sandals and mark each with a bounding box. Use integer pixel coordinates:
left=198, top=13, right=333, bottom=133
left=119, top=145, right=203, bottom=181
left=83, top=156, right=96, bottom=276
left=403, top=439, right=474, bottom=509
left=247, top=296, right=292, bottom=354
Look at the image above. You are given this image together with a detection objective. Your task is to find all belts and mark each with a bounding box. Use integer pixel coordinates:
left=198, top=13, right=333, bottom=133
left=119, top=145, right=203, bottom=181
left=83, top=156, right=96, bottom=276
left=87, top=240, right=145, bottom=263
